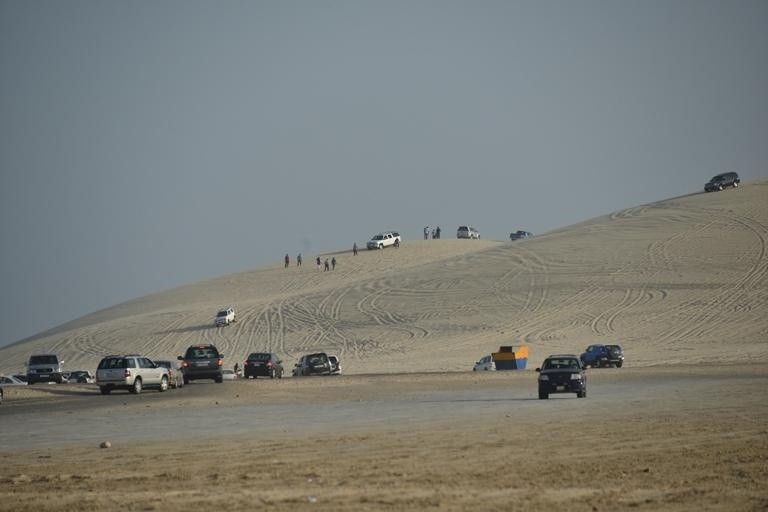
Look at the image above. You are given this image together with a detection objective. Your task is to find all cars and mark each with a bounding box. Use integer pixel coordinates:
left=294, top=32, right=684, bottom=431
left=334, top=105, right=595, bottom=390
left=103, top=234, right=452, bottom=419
left=153, top=360, right=185, bottom=390
left=0, top=370, right=96, bottom=395
left=244, top=352, right=284, bottom=379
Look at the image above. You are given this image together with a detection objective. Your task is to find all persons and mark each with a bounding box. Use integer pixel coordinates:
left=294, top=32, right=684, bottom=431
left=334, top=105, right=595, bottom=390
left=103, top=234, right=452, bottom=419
left=234, top=362, right=239, bottom=373
left=285, top=253, right=290, bottom=268
left=297, top=253, right=302, bottom=267
left=316, top=256, right=336, bottom=271
left=352, top=241, right=359, bottom=256
left=394, top=238, right=399, bottom=248
left=424, top=226, right=440, bottom=240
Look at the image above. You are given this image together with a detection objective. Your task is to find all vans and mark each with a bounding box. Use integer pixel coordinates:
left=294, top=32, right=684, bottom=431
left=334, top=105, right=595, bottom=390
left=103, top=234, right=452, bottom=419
left=215, top=305, right=236, bottom=328
left=456, top=224, right=480, bottom=239
left=473, top=355, right=496, bottom=371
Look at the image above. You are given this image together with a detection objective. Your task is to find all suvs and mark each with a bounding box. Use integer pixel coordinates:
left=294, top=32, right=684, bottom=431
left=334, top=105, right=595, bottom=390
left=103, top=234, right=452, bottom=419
left=703, top=171, right=741, bottom=193
left=292, top=352, right=342, bottom=376
left=177, top=343, right=225, bottom=385
left=95, top=353, right=171, bottom=395
left=24, top=352, right=65, bottom=386
left=536, top=353, right=588, bottom=400
left=580, top=344, right=625, bottom=368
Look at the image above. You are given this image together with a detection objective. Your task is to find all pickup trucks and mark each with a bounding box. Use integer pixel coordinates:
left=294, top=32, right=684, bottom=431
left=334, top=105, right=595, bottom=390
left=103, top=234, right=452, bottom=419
left=509, top=230, right=531, bottom=241
left=366, top=231, right=401, bottom=250
